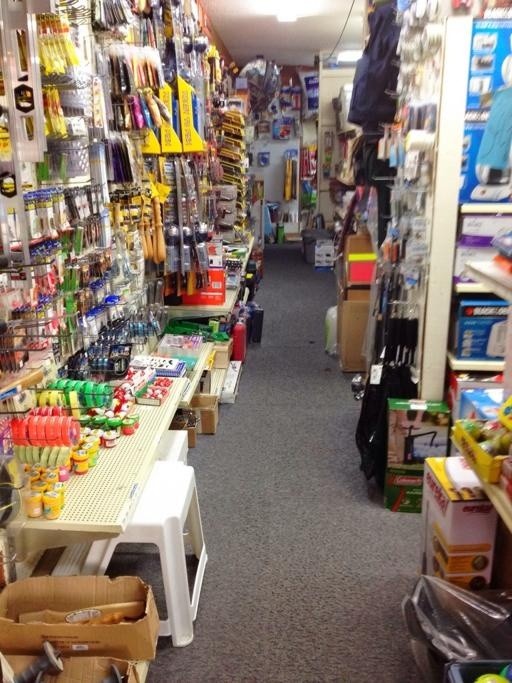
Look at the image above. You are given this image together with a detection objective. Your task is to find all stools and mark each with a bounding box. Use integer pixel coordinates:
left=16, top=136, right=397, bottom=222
left=82, top=457, right=209, bottom=649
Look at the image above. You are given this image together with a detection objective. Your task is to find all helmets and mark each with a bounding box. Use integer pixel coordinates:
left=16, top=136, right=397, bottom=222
left=238, top=59, right=282, bottom=112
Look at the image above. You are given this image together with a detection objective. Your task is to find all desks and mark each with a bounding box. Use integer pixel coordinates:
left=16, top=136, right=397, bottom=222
left=2, top=336, right=213, bottom=562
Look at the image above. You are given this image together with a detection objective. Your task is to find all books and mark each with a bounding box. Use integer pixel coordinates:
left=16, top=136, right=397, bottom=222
left=131, top=357, right=187, bottom=377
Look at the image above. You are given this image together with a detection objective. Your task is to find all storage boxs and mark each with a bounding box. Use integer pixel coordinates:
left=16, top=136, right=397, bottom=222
left=168, top=336, right=236, bottom=449
left=0, top=573, right=160, bottom=683
left=336, top=18, right=511, bottom=589
